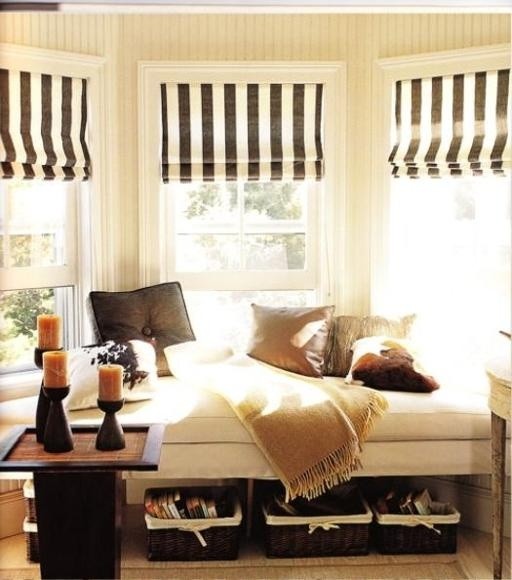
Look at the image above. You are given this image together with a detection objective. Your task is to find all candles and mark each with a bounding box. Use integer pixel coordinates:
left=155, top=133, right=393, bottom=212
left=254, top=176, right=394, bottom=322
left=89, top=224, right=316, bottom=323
left=95, top=360, right=124, bottom=401
left=42, top=350, right=70, bottom=387
left=37, top=313, right=64, bottom=351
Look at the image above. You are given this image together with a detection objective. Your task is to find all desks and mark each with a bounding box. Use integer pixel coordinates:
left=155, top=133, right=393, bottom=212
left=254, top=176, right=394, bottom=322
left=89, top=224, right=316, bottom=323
left=0, top=422, right=164, bottom=580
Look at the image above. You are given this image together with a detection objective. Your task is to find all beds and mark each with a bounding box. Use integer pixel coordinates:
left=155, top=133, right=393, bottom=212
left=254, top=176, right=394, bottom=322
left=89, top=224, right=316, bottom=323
left=1, top=345, right=512, bottom=483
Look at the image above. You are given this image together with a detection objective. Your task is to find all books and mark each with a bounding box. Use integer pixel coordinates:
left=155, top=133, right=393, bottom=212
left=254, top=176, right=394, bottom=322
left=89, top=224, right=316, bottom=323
left=261, top=489, right=301, bottom=518
left=375, top=489, right=455, bottom=515
left=306, top=494, right=348, bottom=516
left=144, top=488, right=218, bottom=519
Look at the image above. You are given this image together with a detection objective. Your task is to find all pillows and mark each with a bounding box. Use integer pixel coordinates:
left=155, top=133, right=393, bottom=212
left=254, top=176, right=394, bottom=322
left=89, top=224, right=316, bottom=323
left=65, top=341, right=158, bottom=411
left=323, top=311, right=417, bottom=379
left=248, top=301, right=335, bottom=379
left=89, top=282, right=198, bottom=353
left=346, top=336, right=439, bottom=392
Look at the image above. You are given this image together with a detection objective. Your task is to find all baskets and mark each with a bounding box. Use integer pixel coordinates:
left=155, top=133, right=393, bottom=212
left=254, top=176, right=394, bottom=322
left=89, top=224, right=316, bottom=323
left=259, top=486, right=374, bottom=558
left=363, top=480, right=462, bottom=554
left=143, top=485, right=244, bottom=561
left=23, top=480, right=41, bottom=563
